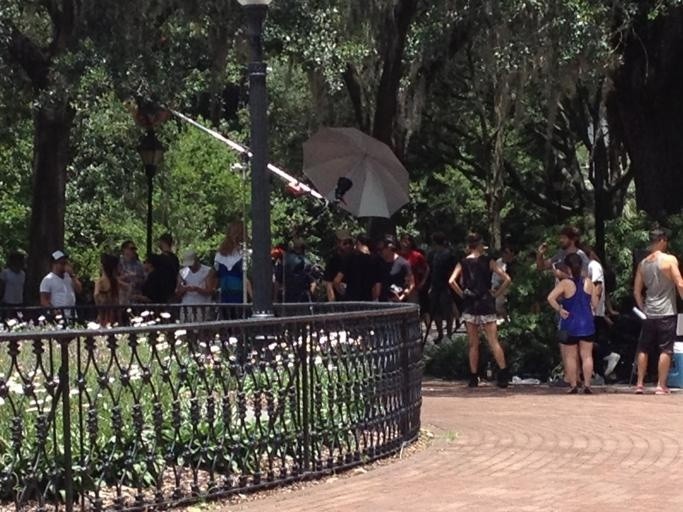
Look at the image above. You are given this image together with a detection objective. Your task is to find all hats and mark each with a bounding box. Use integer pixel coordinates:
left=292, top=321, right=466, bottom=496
left=46, top=247, right=66, bottom=266
left=333, top=229, right=352, bottom=242
left=100, top=252, right=119, bottom=268
left=156, top=234, right=172, bottom=244
left=182, top=251, right=196, bottom=267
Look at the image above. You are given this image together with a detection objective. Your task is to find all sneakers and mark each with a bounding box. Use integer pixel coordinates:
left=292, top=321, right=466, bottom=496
left=655, top=385, right=672, bottom=395
left=566, top=382, right=593, bottom=394
left=604, top=351, right=621, bottom=376
left=633, top=385, right=646, bottom=394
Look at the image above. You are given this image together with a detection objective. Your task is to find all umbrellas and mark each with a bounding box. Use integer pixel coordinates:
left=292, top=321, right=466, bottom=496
left=302, top=126, right=410, bottom=220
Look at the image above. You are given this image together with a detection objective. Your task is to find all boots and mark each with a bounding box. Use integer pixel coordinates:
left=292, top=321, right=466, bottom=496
left=468, top=368, right=509, bottom=388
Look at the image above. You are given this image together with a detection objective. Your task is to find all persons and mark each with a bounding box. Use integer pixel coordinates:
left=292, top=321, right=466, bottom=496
left=633, top=230, right=683, bottom=395
left=2, top=251, right=84, bottom=322
left=271, top=230, right=510, bottom=389
left=546, top=253, right=602, bottom=394
left=579, top=244, right=621, bottom=386
left=537, top=227, right=590, bottom=387
left=94, top=234, right=217, bottom=326
left=216, top=221, right=252, bottom=320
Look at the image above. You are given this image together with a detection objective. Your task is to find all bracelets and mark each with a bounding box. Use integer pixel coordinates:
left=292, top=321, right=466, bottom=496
left=557, top=304, right=563, bottom=313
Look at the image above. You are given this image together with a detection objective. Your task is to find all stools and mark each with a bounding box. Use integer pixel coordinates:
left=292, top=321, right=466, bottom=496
left=667, top=352, right=683, bottom=389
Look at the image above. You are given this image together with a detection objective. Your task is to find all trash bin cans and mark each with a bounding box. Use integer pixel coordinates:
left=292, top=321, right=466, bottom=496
left=664, top=341, right=683, bottom=388
